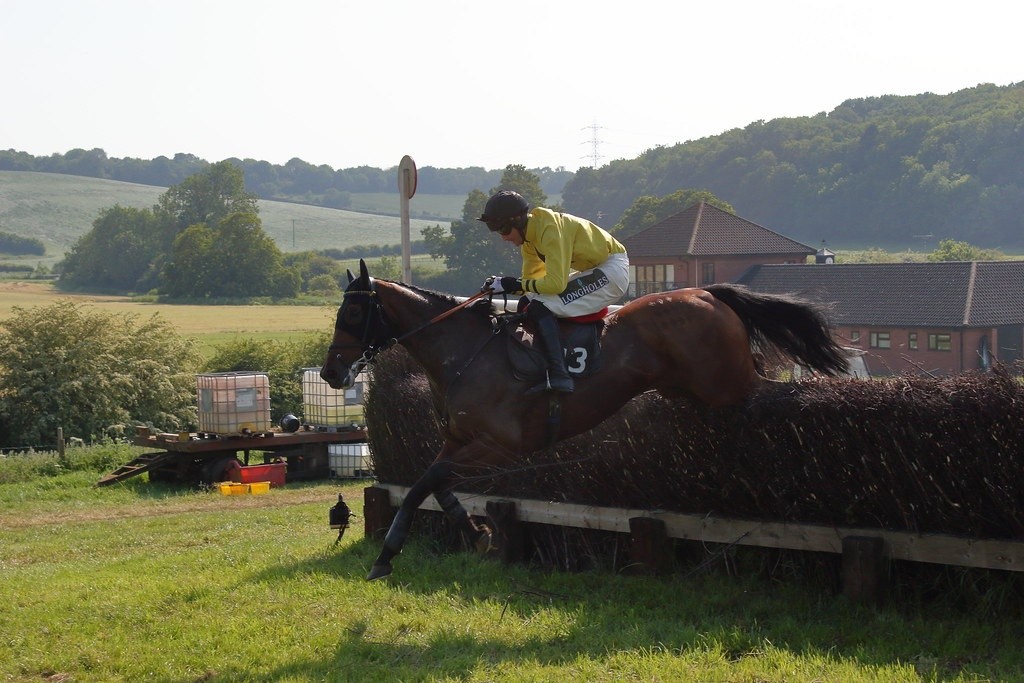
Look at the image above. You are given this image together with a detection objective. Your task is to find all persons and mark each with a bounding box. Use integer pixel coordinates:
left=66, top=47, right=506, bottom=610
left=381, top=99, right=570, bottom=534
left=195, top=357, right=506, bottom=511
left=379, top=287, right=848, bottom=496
left=477, top=191, right=630, bottom=395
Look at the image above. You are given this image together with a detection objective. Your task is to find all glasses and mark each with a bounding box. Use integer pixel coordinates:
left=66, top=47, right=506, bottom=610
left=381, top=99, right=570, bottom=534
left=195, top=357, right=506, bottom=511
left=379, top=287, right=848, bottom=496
left=496, top=223, right=513, bottom=235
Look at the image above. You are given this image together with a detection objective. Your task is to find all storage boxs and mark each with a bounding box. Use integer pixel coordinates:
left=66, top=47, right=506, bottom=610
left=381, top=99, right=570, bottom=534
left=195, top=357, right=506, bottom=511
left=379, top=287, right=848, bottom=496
left=218, top=461, right=288, bottom=496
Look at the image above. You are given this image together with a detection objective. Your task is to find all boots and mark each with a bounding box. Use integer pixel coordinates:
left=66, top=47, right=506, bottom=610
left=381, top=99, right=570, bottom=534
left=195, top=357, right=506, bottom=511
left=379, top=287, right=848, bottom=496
left=523, top=314, right=578, bottom=395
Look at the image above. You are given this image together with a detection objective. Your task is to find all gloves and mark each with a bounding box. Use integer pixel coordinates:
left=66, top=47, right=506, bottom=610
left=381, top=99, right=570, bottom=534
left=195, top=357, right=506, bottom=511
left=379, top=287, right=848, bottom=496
left=481, top=275, right=519, bottom=296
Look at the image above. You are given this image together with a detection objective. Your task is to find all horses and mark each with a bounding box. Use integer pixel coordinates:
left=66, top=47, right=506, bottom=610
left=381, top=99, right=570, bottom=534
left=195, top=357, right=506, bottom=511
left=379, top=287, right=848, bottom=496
left=319, top=257, right=857, bottom=584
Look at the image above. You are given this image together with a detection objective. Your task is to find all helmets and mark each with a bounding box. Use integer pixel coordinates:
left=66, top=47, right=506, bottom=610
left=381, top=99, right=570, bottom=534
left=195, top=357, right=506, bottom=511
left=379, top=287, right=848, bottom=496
left=476, top=190, right=529, bottom=232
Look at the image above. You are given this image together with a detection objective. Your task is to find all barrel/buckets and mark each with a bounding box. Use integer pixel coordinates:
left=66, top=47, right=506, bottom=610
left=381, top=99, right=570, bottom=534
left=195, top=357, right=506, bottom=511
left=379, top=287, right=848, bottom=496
left=221, top=482, right=241, bottom=495
left=250, top=481, right=271, bottom=494
left=280, top=413, right=300, bottom=432
left=229, top=484, right=250, bottom=495
left=179, top=431, right=189, bottom=443
